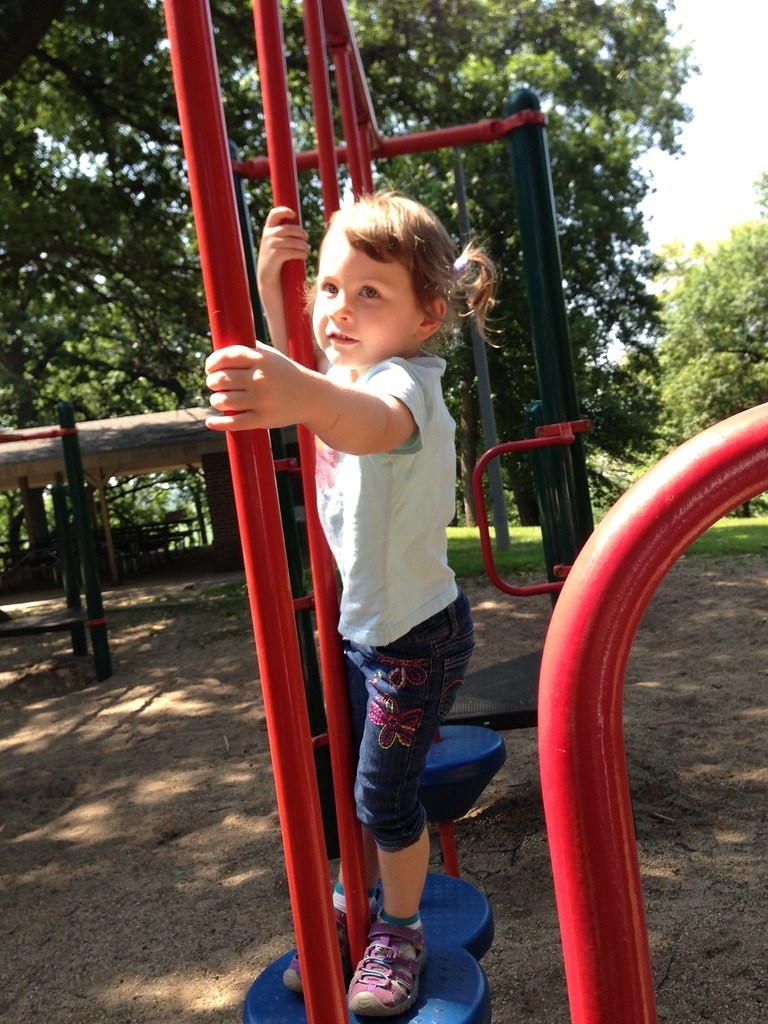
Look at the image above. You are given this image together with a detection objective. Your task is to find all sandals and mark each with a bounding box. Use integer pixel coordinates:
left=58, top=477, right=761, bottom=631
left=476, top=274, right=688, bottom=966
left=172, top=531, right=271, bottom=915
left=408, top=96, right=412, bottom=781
left=283, top=891, right=380, bottom=992
left=348, top=925, right=424, bottom=1017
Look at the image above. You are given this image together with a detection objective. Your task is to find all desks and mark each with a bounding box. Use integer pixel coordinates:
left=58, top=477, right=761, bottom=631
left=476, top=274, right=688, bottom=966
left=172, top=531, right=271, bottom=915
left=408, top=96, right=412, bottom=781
left=0, top=518, right=198, bottom=590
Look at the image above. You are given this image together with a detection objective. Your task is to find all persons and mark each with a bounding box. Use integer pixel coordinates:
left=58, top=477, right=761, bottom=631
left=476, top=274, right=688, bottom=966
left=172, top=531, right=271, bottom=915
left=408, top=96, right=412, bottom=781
left=202, top=191, right=503, bottom=1019
